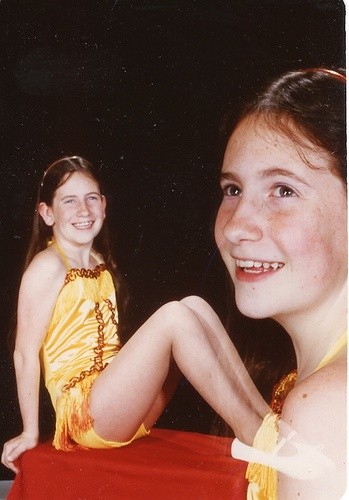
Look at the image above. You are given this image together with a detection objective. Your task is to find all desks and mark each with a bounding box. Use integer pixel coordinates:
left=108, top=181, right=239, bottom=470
left=7, top=428, right=248, bottom=500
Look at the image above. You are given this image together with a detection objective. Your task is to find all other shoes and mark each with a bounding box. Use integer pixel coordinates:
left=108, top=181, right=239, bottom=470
left=231, top=420, right=329, bottom=480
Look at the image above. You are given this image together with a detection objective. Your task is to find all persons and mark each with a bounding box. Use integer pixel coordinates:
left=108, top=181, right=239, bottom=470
left=215, top=67, right=347, bottom=500
left=2, top=155, right=333, bottom=481
